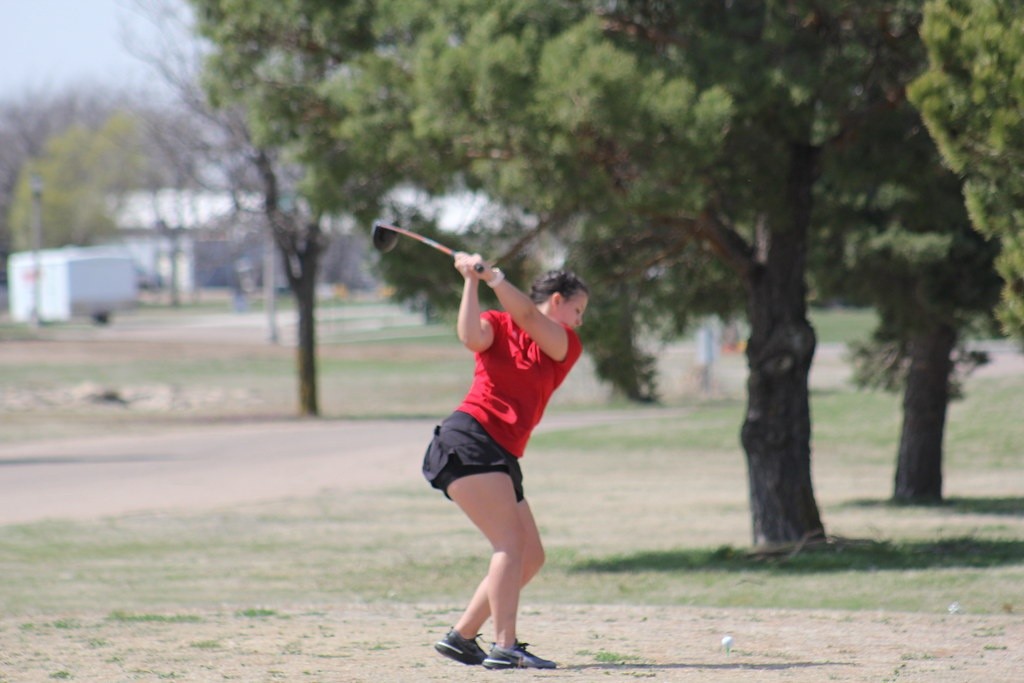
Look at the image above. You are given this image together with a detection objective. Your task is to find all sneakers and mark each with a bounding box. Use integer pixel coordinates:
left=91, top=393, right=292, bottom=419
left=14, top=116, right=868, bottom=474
left=434, top=626, right=488, bottom=666
left=482, top=639, right=556, bottom=671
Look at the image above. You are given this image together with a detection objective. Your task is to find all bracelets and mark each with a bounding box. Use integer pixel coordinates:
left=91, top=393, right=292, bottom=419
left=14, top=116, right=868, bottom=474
left=485, top=264, right=505, bottom=288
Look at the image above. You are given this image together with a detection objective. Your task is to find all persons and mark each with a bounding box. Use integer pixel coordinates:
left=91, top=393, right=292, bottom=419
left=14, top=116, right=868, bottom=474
left=420, top=252, right=591, bottom=672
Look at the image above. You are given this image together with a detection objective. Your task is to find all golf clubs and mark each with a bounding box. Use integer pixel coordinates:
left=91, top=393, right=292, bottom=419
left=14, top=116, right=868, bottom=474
left=372, top=224, right=484, bottom=274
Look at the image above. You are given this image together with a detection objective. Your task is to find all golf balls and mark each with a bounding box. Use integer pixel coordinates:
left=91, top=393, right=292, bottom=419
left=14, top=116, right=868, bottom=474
left=722, top=637, right=734, bottom=648
